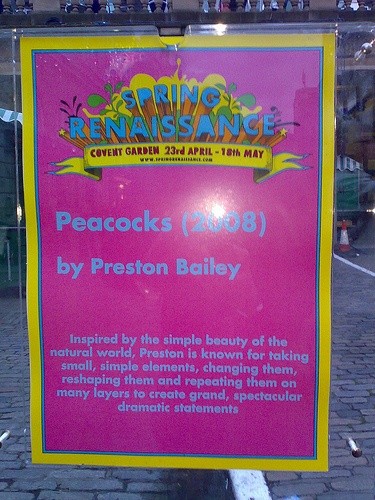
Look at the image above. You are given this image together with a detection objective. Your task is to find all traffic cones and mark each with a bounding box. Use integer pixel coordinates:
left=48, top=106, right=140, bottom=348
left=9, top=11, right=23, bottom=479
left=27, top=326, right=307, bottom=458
left=337, top=220, right=352, bottom=253
